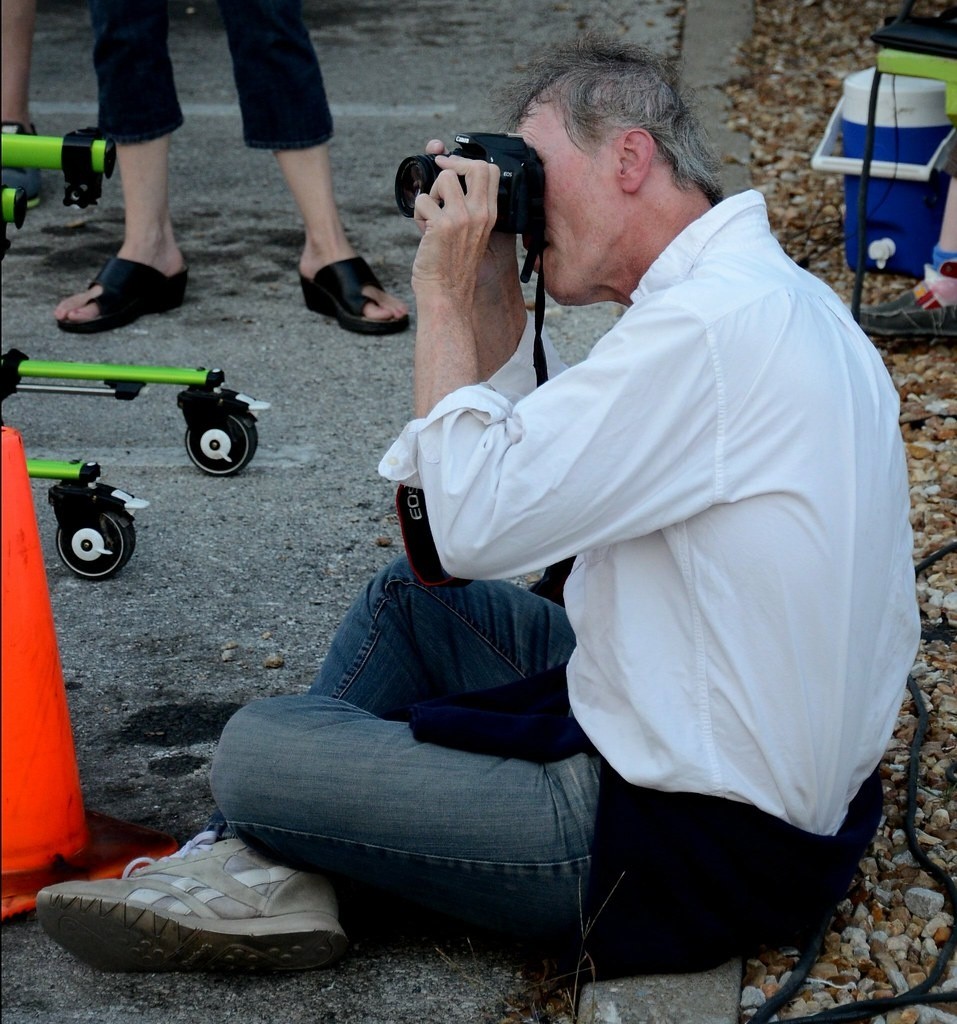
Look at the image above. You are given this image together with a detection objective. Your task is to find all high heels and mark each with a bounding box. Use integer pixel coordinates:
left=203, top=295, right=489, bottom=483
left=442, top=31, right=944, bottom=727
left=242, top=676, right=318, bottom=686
left=300, top=257, right=409, bottom=334
left=57, top=256, right=189, bottom=334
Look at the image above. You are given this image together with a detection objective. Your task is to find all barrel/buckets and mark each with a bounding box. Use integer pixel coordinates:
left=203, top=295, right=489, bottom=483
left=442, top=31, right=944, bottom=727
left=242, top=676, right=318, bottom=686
left=809, top=65, right=954, bottom=279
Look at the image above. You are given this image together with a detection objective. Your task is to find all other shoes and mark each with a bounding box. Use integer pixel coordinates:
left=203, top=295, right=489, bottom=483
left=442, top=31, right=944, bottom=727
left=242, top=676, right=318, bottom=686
left=854, top=279, right=957, bottom=337
left=0, top=120, right=42, bottom=208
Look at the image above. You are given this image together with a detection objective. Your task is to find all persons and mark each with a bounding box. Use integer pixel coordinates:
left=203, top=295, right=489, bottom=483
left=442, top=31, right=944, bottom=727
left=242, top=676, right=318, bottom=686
left=36, top=34, right=920, bottom=983
left=0, top=0, right=411, bottom=336
left=852, top=144, right=957, bottom=346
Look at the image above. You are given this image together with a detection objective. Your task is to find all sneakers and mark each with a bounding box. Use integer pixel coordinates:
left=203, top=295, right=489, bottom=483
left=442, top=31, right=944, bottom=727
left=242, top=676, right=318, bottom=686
left=36, top=831, right=348, bottom=974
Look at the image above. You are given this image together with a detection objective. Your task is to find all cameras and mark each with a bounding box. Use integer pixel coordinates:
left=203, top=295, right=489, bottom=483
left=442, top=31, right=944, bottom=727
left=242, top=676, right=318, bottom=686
left=394, top=134, right=545, bottom=234
